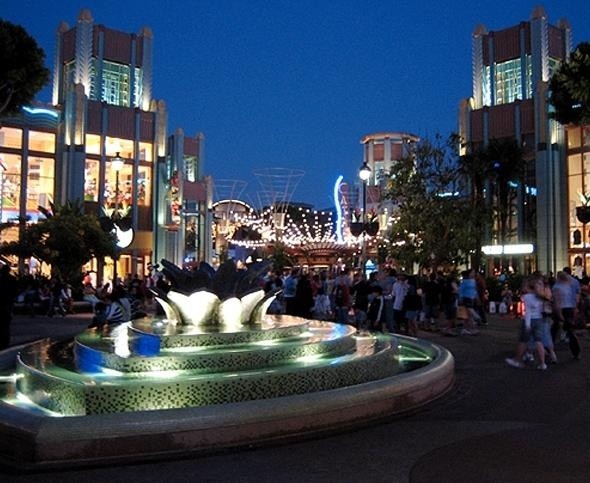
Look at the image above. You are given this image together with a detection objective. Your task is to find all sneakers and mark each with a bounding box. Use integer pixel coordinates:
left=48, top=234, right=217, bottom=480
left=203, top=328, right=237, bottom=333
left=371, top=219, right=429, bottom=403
left=506, top=351, right=557, bottom=370
left=446, top=327, right=480, bottom=336
left=474, top=319, right=488, bottom=326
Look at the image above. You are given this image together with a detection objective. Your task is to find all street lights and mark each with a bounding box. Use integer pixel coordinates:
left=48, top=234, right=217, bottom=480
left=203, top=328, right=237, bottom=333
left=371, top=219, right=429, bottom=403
left=358, top=160, right=374, bottom=279
left=108, top=148, right=125, bottom=301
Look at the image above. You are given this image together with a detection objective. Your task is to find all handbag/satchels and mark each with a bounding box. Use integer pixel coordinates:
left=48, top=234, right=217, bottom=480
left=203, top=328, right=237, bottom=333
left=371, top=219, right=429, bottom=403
left=403, top=294, right=423, bottom=311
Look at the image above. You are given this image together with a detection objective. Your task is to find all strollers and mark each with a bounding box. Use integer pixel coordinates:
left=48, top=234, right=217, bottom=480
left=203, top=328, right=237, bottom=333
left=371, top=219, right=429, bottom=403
left=365, top=295, right=386, bottom=328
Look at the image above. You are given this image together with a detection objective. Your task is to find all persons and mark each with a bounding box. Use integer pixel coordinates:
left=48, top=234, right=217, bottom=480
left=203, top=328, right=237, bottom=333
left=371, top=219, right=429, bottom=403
left=0, top=263, right=18, bottom=351
left=19, top=271, right=159, bottom=329
left=252, top=256, right=589, bottom=371
